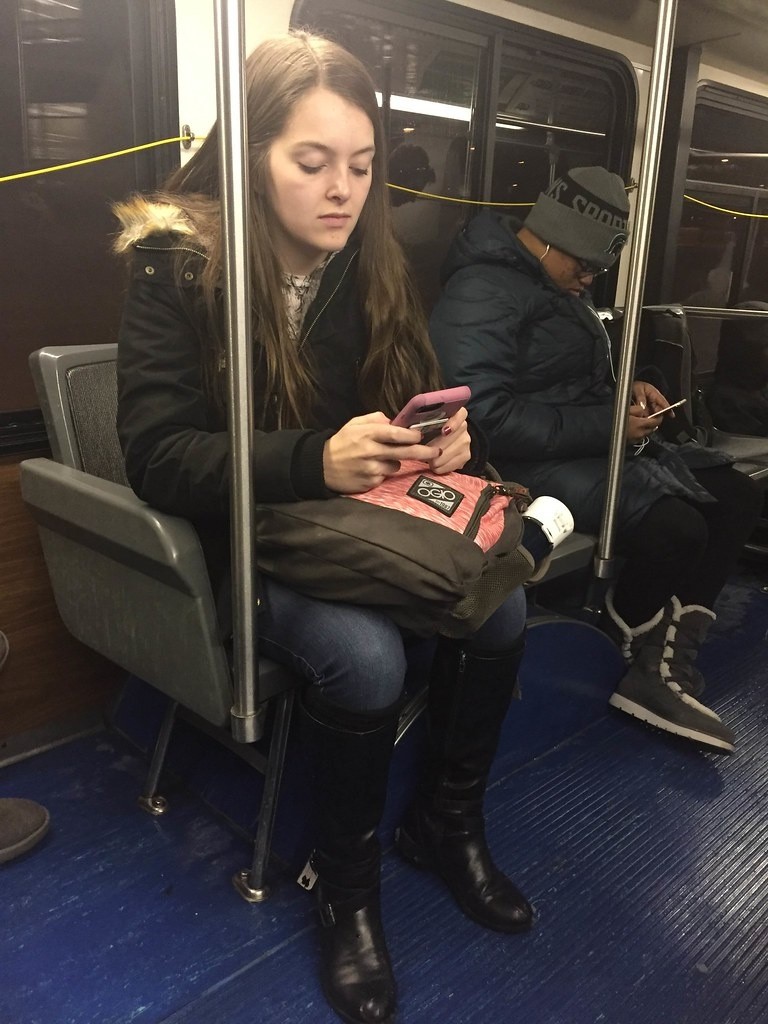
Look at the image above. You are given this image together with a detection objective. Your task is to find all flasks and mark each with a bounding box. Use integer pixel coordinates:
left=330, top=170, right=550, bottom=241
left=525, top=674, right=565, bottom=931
left=516, top=496, right=577, bottom=566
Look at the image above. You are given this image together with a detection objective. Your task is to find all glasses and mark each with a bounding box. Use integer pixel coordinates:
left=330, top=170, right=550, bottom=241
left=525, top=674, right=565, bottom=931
left=569, top=256, right=607, bottom=277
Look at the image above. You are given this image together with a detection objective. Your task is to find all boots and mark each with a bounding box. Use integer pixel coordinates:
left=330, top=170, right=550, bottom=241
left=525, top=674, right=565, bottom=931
left=396, top=628, right=534, bottom=937
left=602, top=596, right=738, bottom=754
left=297, top=683, right=408, bottom=1022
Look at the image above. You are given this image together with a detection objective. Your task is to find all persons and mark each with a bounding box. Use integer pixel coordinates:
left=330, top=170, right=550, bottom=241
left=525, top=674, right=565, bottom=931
left=428, top=164, right=736, bottom=750
left=110, top=26, right=535, bottom=1023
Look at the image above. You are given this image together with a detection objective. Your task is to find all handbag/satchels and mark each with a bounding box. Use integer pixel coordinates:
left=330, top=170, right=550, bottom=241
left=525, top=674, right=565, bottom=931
left=243, top=453, right=553, bottom=643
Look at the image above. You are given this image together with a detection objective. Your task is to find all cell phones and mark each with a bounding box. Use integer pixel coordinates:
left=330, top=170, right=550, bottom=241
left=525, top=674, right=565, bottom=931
left=392, top=387, right=472, bottom=443
left=647, top=399, right=687, bottom=419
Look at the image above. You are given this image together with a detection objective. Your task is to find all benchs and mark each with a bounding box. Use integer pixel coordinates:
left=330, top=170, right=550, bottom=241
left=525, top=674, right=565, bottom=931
left=21, top=300, right=768, bottom=902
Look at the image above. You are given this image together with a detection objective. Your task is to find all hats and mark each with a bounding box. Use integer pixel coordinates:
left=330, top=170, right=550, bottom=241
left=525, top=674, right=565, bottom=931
left=525, top=164, right=631, bottom=266
